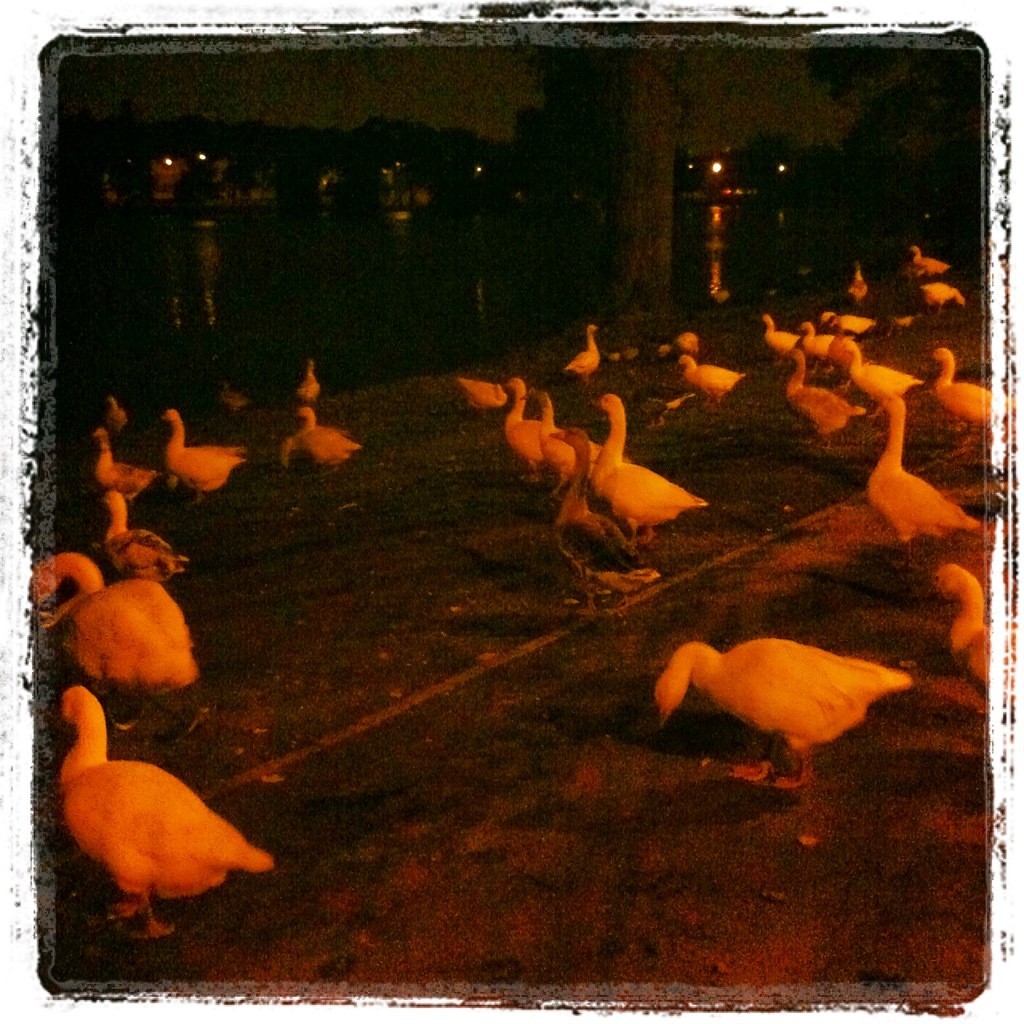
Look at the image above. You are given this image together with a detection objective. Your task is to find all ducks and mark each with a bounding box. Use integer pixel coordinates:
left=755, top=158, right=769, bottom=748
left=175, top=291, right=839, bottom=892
left=564, top=324, right=601, bottom=383
left=218, top=381, right=252, bottom=411
left=295, top=356, right=321, bottom=403
left=31, top=552, right=199, bottom=694
left=499, top=378, right=546, bottom=482
left=52, top=682, right=273, bottom=937
left=921, top=562, right=989, bottom=698
left=910, top=245, right=952, bottom=274
left=919, top=281, right=965, bottom=315
left=453, top=373, right=506, bottom=412
left=926, top=347, right=992, bottom=426
left=519, top=387, right=633, bottom=497
left=607, top=332, right=700, bottom=361
left=759, top=310, right=924, bottom=443
left=587, top=393, right=709, bottom=545
left=653, top=637, right=913, bottom=785
left=279, top=428, right=362, bottom=469
left=160, top=409, right=248, bottom=502
left=295, top=405, right=317, bottom=438
left=849, top=260, right=869, bottom=303
left=88, top=427, right=161, bottom=500
left=543, top=427, right=661, bottom=616
left=96, top=490, right=190, bottom=586
left=674, top=354, right=747, bottom=402
left=866, top=394, right=982, bottom=562
